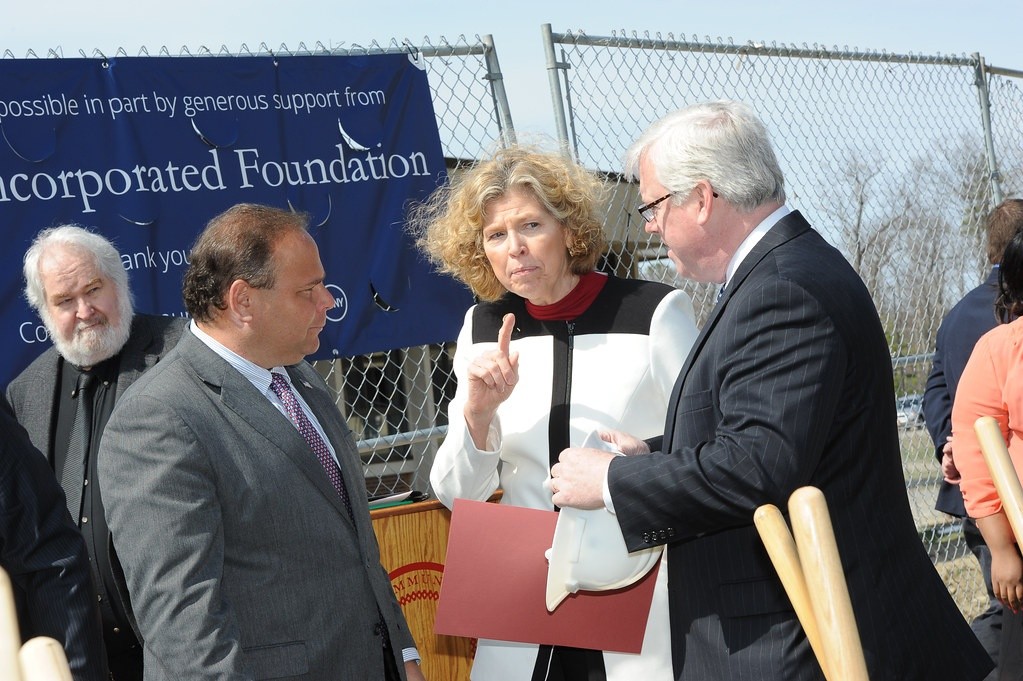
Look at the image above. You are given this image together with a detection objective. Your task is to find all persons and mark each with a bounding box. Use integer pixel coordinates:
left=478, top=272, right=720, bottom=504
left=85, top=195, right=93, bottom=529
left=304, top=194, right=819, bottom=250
left=5, top=225, right=190, bottom=681
left=427, top=151, right=702, bottom=680
left=96, top=203, right=423, bottom=680
left=921, top=202, right=1023, bottom=681
left=0, top=389, right=112, bottom=681
left=552, top=103, right=996, bottom=681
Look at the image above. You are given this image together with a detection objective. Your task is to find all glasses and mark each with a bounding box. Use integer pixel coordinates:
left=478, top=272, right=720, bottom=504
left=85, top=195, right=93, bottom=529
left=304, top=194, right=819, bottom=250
left=637, top=186, right=719, bottom=223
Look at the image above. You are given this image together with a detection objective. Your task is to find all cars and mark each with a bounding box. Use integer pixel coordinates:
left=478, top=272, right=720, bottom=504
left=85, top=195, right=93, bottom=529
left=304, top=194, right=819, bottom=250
left=896, top=394, right=925, bottom=431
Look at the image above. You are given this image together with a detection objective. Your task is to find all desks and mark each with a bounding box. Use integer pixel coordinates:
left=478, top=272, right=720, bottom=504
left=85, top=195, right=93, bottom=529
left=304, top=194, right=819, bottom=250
left=369, top=489, right=504, bottom=681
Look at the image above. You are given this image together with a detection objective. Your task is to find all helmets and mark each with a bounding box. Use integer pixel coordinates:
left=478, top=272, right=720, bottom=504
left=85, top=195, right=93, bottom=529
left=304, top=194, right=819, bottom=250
left=546, top=430, right=664, bottom=613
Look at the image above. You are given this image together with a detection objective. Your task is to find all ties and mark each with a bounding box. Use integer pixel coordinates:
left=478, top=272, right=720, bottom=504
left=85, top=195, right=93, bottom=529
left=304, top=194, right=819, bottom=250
left=62, top=372, right=97, bottom=526
left=715, top=281, right=726, bottom=307
left=270, top=371, right=356, bottom=526
